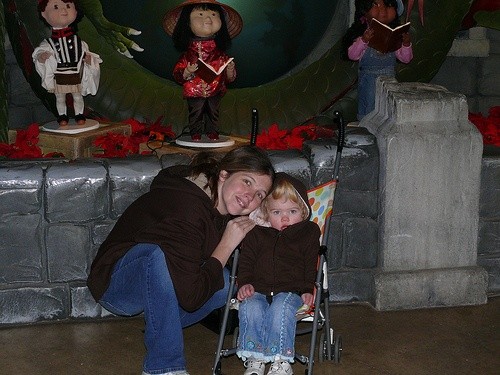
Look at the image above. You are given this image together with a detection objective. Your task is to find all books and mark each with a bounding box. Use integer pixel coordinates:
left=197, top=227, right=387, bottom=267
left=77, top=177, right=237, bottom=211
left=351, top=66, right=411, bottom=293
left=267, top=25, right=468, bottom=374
left=367, top=18, right=410, bottom=54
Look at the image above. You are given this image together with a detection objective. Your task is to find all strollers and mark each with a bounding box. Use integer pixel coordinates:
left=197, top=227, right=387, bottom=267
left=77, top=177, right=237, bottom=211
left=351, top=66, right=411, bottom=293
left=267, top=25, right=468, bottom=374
left=210, top=109, right=343, bottom=375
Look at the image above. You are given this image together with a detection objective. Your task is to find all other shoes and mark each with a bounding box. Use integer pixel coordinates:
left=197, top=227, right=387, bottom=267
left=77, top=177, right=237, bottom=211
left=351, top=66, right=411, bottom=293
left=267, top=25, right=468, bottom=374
left=243, top=358, right=294, bottom=375
left=141, top=370, right=191, bottom=375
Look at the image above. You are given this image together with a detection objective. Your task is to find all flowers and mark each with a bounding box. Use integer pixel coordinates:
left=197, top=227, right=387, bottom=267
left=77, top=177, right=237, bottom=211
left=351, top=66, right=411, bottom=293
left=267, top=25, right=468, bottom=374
left=468, top=106, right=500, bottom=147
left=247, top=123, right=335, bottom=152
left=91, top=118, right=176, bottom=157
left=0, top=121, right=65, bottom=160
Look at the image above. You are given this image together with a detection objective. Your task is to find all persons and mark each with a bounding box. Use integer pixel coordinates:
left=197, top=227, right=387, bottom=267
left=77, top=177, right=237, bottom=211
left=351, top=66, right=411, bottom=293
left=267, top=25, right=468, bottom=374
left=86, top=145, right=274, bottom=375
left=234, top=172, right=321, bottom=375
left=32, top=0, right=102, bottom=127
left=341, top=0, right=412, bottom=121
left=161, top=0, right=243, bottom=143
